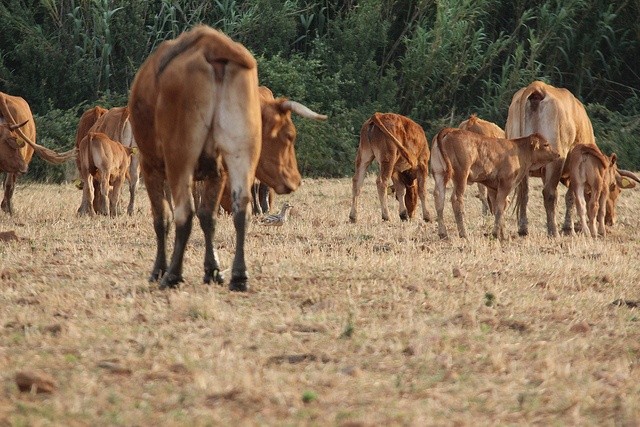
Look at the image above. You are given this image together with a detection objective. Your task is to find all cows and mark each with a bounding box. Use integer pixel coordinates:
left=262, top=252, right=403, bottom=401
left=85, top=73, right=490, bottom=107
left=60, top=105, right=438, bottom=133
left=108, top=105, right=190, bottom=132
left=505, top=81, right=640, bottom=238
left=350, top=112, right=432, bottom=224
left=458, top=116, right=510, bottom=215
left=430, top=128, right=560, bottom=242
left=568, top=144, right=617, bottom=237
left=128, top=26, right=327, bottom=293
left=78, top=133, right=138, bottom=216
left=0, top=91, right=77, bottom=216
left=77, top=106, right=108, bottom=216
left=88, top=107, right=140, bottom=215
left=158, top=87, right=274, bottom=219
